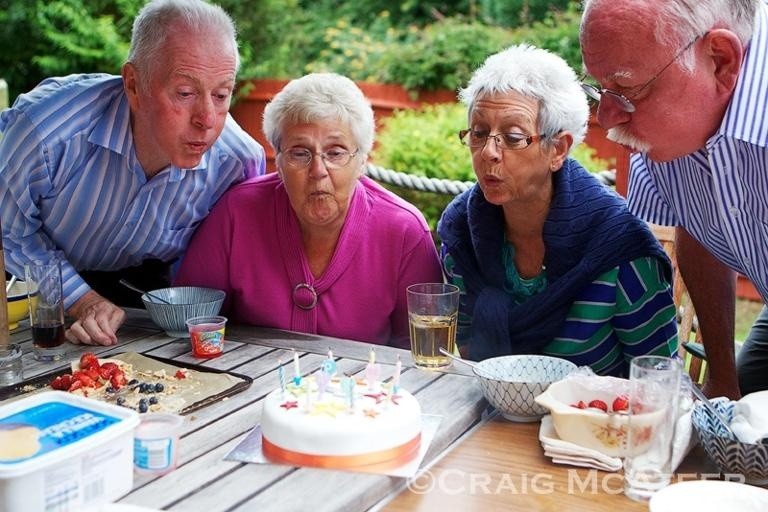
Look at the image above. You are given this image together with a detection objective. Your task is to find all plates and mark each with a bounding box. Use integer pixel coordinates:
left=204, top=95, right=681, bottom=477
left=648, top=480, right=767, bottom=511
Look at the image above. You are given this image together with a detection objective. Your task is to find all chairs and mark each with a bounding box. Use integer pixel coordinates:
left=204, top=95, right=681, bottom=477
left=646, top=223, right=703, bottom=381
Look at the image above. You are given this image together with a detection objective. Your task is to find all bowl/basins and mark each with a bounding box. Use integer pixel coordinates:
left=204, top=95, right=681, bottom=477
left=691, top=401, right=768, bottom=486
left=472, top=356, right=578, bottom=424
left=5, top=280, right=39, bottom=331
left=140, top=286, right=226, bottom=338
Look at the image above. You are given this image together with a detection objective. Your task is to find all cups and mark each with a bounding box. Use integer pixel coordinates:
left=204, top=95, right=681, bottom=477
left=134, top=413, right=184, bottom=477
left=0, top=343, right=24, bottom=390
left=186, top=317, right=228, bottom=359
left=624, top=355, right=681, bottom=503
left=406, top=283, right=460, bottom=371
left=24, top=260, right=66, bottom=362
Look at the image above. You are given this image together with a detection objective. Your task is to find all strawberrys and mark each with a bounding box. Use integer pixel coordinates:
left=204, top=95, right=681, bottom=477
left=52, top=354, right=124, bottom=398
left=572, top=392, right=649, bottom=415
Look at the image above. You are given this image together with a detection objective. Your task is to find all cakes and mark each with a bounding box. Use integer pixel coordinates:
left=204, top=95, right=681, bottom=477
left=259, top=344, right=422, bottom=476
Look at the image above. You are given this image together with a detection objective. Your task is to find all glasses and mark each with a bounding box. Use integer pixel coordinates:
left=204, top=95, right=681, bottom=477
left=578, top=36, right=699, bottom=112
left=460, top=128, right=546, bottom=150
left=275, top=147, right=359, bottom=169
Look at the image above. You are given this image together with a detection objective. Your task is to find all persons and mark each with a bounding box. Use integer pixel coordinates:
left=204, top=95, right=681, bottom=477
left=0, top=1, right=267, bottom=346
left=172, top=72, right=444, bottom=350
left=578, top=0, right=768, bottom=402
left=436, top=42, right=678, bottom=379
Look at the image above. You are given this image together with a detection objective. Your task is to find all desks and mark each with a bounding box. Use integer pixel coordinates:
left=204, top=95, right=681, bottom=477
left=367, top=408, right=768, bottom=512
left=0, top=304, right=488, bottom=512
